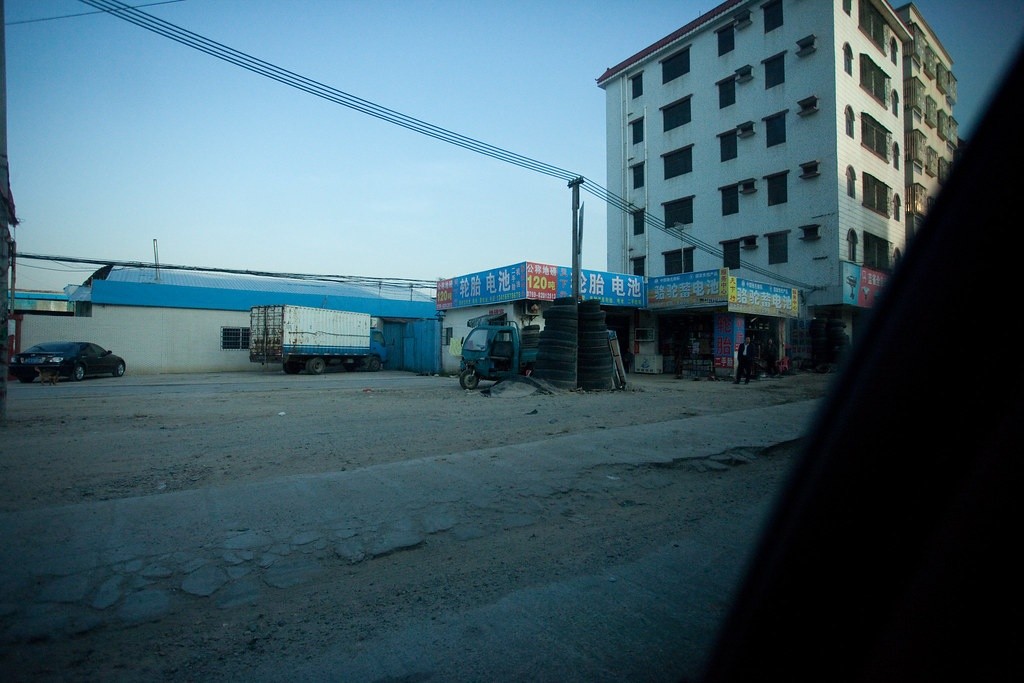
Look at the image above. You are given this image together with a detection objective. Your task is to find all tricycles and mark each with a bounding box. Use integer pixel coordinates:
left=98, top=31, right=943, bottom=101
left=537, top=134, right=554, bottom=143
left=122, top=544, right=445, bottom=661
left=459, top=321, right=539, bottom=389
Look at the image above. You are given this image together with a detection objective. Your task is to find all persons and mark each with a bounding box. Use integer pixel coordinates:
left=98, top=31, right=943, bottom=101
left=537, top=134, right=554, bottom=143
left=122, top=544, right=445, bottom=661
left=764, top=338, right=777, bottom=352
left=733, top=336, right=755, bottom=384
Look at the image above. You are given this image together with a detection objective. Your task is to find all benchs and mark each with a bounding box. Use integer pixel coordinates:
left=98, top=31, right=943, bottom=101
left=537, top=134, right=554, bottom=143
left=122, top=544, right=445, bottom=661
left=490, top=341, right=513, bottom=360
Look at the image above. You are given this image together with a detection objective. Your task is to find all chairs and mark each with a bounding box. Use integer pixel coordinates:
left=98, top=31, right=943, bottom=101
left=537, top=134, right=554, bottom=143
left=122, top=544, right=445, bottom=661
left=775, top=356, right=790, bottom=374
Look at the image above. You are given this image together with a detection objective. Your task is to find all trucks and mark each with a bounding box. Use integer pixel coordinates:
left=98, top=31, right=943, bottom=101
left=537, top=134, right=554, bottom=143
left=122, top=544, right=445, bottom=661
left=249, top=304, right=388, bottom=376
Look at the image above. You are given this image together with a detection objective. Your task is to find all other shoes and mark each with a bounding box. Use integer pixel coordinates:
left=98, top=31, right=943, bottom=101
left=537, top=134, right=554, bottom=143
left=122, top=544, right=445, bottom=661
left=745, top=381, right=748, bottom=382
left=733, top=381, right=739, bottom=384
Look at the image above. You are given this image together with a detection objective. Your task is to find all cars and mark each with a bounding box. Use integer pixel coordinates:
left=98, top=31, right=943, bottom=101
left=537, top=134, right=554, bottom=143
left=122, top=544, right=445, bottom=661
left=7, top=342, right=126, bottom=384
